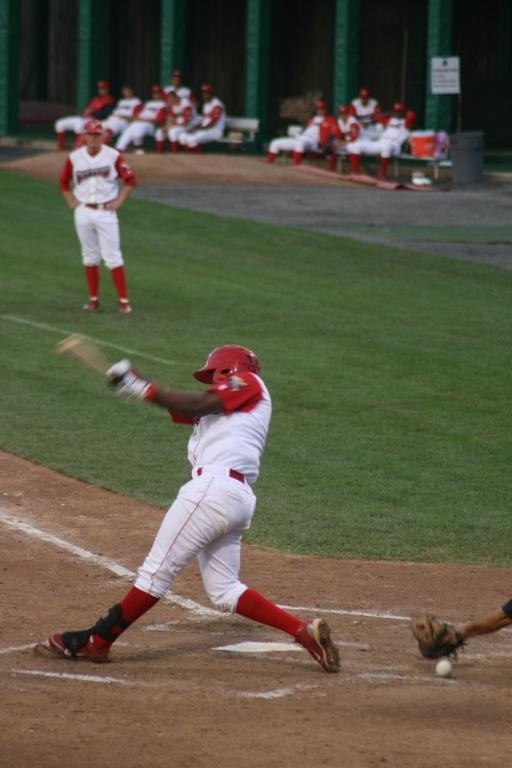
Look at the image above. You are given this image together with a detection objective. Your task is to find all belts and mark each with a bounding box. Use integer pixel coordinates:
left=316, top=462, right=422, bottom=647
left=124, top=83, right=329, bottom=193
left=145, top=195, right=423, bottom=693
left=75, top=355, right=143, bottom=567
left=76, top=203, right=113, bottom=210
left=190, top=465, right=248, bottom=483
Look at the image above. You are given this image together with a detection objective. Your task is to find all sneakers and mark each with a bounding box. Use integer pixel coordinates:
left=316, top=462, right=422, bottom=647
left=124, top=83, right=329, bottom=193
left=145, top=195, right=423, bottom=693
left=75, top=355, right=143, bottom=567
left=118, top=299, right=132, bottom=314
left=49, top=629, right=110, bottom=663
left=294, top=617, right=341, bottom=673
left=82, top=297, right=100, bottom=310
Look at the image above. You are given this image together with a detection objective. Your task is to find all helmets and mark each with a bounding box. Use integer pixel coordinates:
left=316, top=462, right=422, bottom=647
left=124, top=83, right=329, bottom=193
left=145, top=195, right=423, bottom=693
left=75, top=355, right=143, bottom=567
left=194, top=343, right=260, bottom=384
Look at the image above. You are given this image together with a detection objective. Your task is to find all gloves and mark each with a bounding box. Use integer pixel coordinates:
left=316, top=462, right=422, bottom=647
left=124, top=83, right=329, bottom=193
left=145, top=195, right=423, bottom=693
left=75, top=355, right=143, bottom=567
left=105, top=359, right=153, bottom=403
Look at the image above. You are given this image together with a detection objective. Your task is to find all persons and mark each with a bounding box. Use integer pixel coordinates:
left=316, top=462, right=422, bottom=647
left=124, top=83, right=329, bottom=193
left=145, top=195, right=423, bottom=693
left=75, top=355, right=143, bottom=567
left=59, top=117, right=138, bottom=314
left=48, top=330, right=339, bottom=674
left=265, top=89, right=417, bottom=181
left=54, top=70, right=229, bottom=156
left=412, top=600, right=512, bottom=662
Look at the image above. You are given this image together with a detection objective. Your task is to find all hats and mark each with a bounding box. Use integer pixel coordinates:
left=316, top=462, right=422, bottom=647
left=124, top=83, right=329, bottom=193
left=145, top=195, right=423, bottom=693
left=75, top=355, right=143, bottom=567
left=83, top=118, right=104, bottom=135
left=315, top=89, right=404, bottom=114
left=97, top=70, right=212, bottom=98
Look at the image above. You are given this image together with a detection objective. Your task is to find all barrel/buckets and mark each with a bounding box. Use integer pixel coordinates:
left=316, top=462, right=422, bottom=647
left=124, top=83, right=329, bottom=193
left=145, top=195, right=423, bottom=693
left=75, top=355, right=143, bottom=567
left=409, top=129, right=436, bottom=158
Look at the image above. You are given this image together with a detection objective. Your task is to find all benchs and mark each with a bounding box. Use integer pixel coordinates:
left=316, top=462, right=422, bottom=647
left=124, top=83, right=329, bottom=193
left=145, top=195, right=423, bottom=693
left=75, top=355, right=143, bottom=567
left=190, top=113, right=451, bottom=183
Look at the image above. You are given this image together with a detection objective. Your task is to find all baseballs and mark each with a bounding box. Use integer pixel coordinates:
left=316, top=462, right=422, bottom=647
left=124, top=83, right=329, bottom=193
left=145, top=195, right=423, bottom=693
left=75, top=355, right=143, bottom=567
left=436, top=659, right=453, bottom=678
